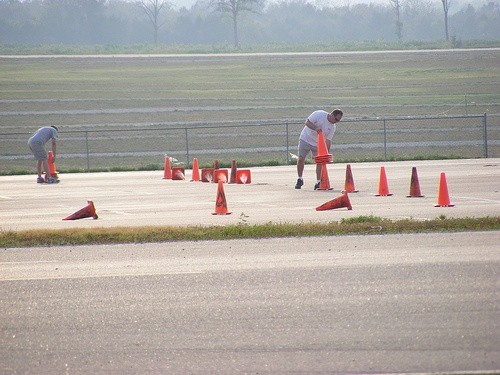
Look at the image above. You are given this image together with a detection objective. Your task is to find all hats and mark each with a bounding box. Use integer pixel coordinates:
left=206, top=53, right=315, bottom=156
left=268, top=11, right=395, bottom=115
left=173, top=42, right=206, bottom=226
left=50, top=125, right=58, bottom=131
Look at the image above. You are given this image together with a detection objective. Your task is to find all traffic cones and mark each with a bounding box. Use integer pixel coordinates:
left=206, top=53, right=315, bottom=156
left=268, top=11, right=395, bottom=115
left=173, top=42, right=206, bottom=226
left=317, top=163, right=334, bottom=191
left=316, top=189, right=352, bottom=211
left=62, top=200, right=99, bottom=220
left=190, top=158, right=202, bottom=182
left=202, top=160, right=228, bottom=183
left=374, top=166, right=392, bottom=197
left=46, top=152, right=59, bottom=181
left=164, top=157, right=186, bottom=180
left=433, top=172, right=455, bottom=207
left=342, top=165, right=359, bottom=194
left=211, top=179, right=235, bottom=216
left=313, top=130, right=334, bottom=164
left=230, top=160, right=251, bottom=184
left=406, top=167, right=425, bottom=198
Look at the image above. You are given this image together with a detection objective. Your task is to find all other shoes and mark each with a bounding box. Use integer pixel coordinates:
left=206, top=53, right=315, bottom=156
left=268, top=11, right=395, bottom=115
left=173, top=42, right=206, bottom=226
left=314, top=181, right=321, bottom=191
left=46, top=177, right=56, bottom=183
left=295, top=178, right=303, bottom=189
left=37, top=177, right=44, bottom=183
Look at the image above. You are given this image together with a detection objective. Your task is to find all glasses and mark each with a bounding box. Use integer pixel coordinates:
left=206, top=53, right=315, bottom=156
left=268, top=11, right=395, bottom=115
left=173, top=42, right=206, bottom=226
left=334, top=116, right=339, bottom=122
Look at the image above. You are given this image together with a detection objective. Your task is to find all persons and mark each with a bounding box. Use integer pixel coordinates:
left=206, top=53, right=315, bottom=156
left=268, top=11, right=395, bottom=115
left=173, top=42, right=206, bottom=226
left=295, top=109, right=343, bottom=190
left=29, top=125, right=60, bottom=183
